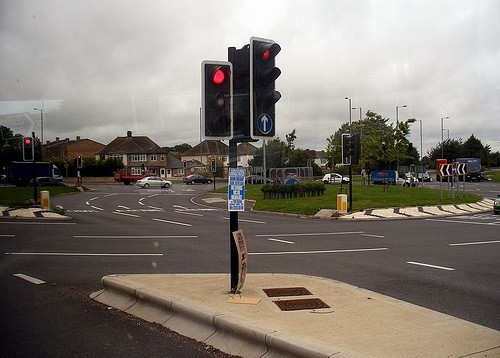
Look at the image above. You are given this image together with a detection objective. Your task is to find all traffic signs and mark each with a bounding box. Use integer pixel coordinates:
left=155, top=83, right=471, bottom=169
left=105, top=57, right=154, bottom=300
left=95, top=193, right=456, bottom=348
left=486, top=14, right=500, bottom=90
left=396, top=105, right=407, bottom=170
left=352, top=107, right=362, bottom=158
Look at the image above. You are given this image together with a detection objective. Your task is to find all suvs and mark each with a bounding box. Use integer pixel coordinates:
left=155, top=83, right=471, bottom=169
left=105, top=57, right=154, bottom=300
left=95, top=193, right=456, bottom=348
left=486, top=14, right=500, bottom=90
left=322, top=173, right=350, bottom=184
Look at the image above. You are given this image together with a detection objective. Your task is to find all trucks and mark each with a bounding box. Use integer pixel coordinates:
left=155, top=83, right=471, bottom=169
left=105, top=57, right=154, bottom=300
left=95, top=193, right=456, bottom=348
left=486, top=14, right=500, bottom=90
left=114, top=168, right=155, bottom=185
left=436, top=158, right=481, bottom=182
left=371, top=169, right=419, bottom=187
left=10, top=161, right=63, bottom=187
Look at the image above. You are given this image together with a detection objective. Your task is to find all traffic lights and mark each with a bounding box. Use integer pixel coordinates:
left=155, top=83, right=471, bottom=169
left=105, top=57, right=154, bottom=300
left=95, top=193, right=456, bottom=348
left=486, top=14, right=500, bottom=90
left=24, top=137, right=33, bottom=160
left=211, top=158, right=216, bottom=173
left=351, top=133, right=360, bottom=165
left=202, top=61, right=234, bottom=141
left=184, top=160, right=186, bottom=167
left=228, top=43, right=260, bottom=142
left=77, top=154, right=83, bottom=168
left=250, top=36, right=281, bottom=139
left=342, top=134, right=354, bottom=164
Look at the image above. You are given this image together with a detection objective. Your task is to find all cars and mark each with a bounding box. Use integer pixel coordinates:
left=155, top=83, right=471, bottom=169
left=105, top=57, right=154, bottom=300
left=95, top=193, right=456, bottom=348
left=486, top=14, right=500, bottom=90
left=494, top=193, right=500, bottom=215
left=135, top=176, right=172, bottom=188
left=29, top=177, right=67, bottom=187
left=183, top=174, right=213, bottom=185
left=245, top=175, right=275, bottom=184
left=417, top=172, right=430, bottom=182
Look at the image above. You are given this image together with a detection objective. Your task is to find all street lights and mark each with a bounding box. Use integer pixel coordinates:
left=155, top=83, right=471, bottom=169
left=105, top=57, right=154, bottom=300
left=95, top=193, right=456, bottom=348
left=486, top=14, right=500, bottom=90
left=413, top=119, right=423, bottom=172
left=345, top=97, right=352, bottom=135
left=34, top=108, right=43, bottom=161
left=441, top=116, right=450, bottom=159
